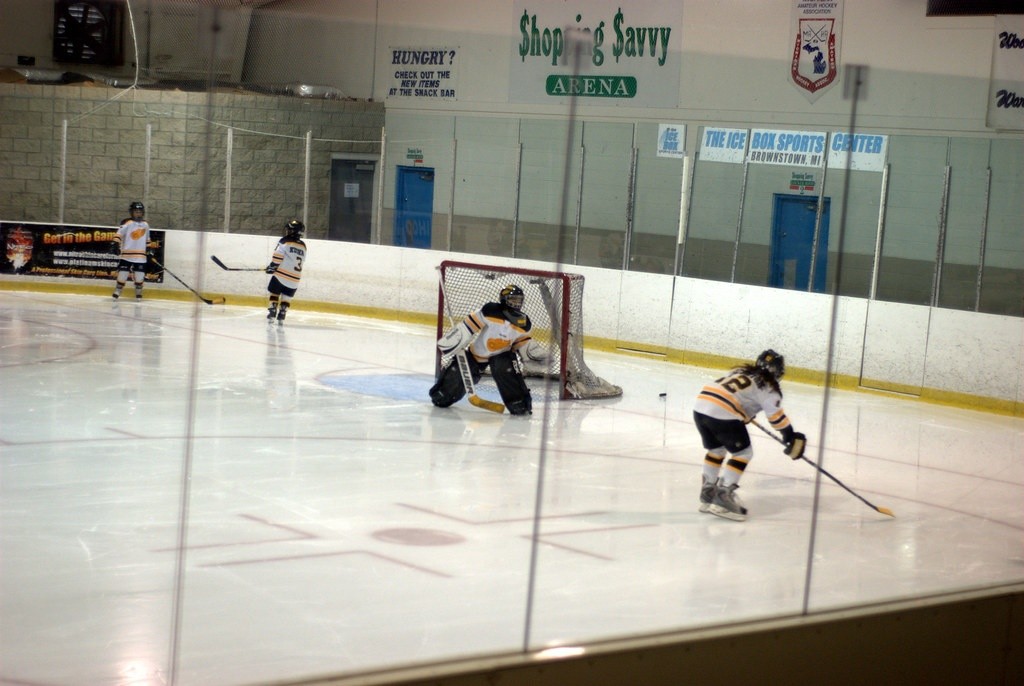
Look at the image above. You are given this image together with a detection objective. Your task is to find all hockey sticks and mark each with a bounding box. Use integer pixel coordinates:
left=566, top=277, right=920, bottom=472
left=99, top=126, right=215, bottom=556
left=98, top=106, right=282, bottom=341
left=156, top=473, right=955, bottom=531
left=210, top=255, right=265, bottom=271
left=436, top=266, right=506, bottom=414
left=751, top=417, right=896, bottom=517
left=151, top=258, right=226, bottom=305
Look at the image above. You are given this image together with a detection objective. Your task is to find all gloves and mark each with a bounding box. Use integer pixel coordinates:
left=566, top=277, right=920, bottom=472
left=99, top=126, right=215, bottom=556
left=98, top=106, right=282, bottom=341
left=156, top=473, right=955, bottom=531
left=784, top=431, right=806, bottom=459
left=146, top=247, right=154, bottom=260
left=266, top=262, right=279, bottom=274
left=109, top=240, right=121, bottom=256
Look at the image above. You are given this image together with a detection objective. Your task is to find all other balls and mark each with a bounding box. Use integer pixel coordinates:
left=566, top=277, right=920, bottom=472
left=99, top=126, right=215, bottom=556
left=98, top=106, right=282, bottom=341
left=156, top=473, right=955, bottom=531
left=658, top=393, right=667, bottom=397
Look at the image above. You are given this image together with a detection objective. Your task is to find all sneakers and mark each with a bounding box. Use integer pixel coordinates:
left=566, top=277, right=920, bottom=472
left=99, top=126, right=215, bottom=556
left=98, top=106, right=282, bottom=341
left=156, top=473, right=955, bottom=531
left=135, top=284, right=142, bottom=301
left=707, top=478, right=748, bottom=521
left=700, top=473, right=720, bottom=512
left=276, top=305, right=288, bottom=325
left=267, top=302, right=278, bottom=322
left=112, top=281, right=125, bottom=301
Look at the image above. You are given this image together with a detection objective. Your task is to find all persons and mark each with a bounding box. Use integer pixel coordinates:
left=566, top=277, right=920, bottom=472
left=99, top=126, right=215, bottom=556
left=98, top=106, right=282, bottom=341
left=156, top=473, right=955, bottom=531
left=110, top=201, right=161, bottom=299
left=693, top=347, right=807, bottom=517
left=429, top=283, right=553, bottom=417
left=265, top=219, right=307, bottom=321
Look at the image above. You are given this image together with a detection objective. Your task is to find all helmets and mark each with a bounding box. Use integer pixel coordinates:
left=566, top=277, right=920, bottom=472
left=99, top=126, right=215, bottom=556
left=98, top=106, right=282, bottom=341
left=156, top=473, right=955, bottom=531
left=500, top=285, right=524, bottom=312
left=289, top=220, right=305, bottom=236
left=129, top=202, right=145, bottom=218
left=756, top=349, right=784, bottom=383
